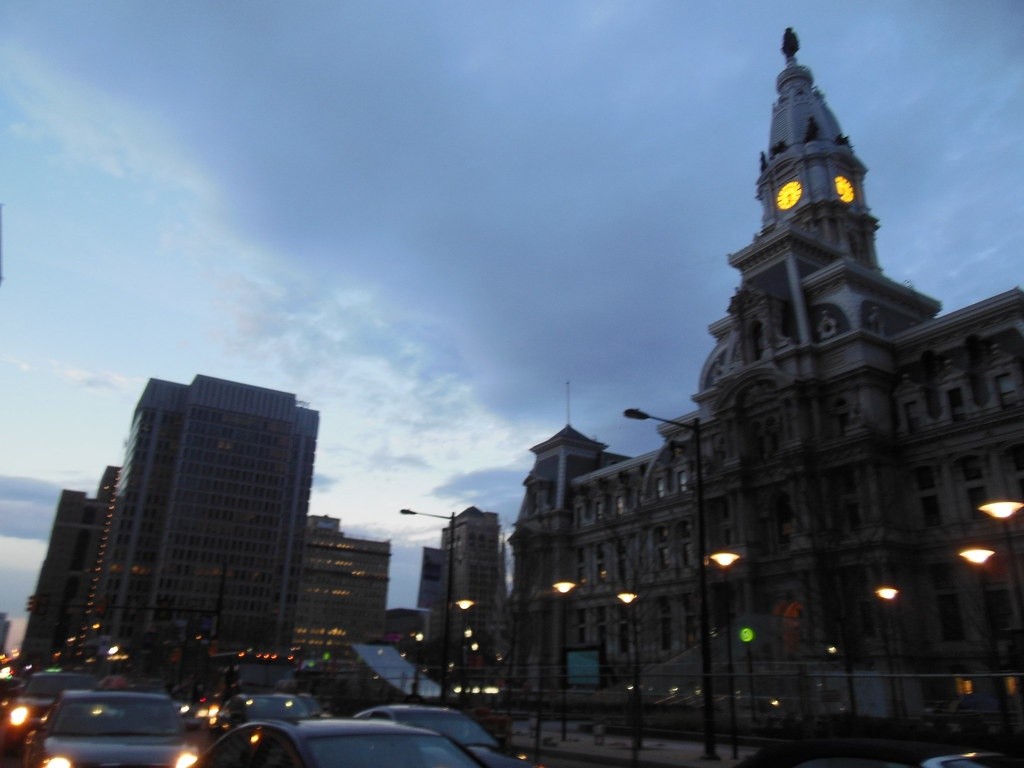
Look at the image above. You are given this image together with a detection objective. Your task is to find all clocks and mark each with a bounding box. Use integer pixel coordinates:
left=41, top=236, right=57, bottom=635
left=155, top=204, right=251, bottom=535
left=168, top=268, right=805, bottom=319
left=777, top=183, right=801, bottom=210
left=836, top=176, right=854, bottom=203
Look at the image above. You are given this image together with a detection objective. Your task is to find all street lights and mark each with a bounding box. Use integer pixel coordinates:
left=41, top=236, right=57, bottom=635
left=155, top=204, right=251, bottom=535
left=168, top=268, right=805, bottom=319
left=973, top=497, right=1024, bottom=634
left=708, top=538, right=741, bottom=759
left=618, top=590, right=644, bottom=748
left=455, top=592, right=476, bottom=686
left=399, top=507, right=458, bottom=705
left=950, top=530, right=1015, bottom=738
left=550, top=573, right=577, bottom=741
left=624, top=407, right=723, bottom=763
left=872, top=582, right=912, bottom=728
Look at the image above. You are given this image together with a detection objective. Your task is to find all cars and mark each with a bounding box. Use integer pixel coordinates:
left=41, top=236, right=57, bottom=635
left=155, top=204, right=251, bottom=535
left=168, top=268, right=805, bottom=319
left=21, top=688, right=202, bottom=767
left=212, top=692, right=320, bottom=738
left=0, top=669, right=98, bottom=753
left=353, top=704, right=546, bottom=768
left=187, top=714, right=484, bottom=768
